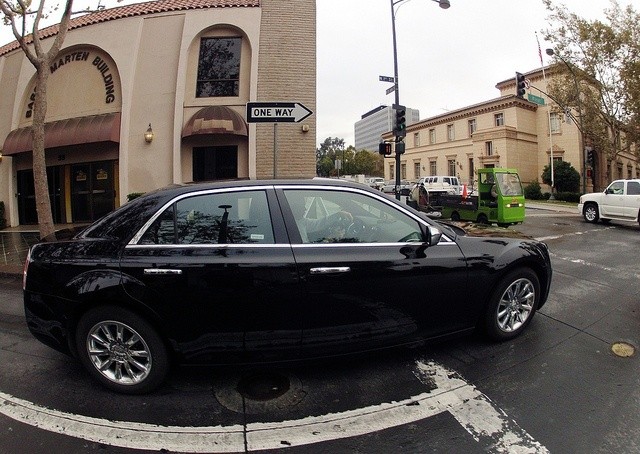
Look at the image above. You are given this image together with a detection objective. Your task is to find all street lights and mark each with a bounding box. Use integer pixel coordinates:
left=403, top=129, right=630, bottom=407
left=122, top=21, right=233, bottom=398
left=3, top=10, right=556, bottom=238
left=391, top=0, right=462, bottom=200
left=546, top=48, right=586, bottom=194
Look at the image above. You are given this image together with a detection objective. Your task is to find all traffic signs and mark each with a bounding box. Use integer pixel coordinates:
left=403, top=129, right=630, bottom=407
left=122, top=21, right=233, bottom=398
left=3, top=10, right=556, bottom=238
left=386, top=84, right=395, bottom=94
left=246, top=101, right=312, bottom=123
left=379, top=75, right=394, bottom=82
left=527, top=92, right=544, bottom=105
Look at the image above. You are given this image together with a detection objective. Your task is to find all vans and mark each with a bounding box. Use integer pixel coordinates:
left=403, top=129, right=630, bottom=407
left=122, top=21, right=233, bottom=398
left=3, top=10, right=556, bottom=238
left=409, top=175, right=460, bottom=196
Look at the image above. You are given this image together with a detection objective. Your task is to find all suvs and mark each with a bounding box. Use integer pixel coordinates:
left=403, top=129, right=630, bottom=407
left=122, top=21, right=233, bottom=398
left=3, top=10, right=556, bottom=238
left=578, top=178, right=640, bottom=225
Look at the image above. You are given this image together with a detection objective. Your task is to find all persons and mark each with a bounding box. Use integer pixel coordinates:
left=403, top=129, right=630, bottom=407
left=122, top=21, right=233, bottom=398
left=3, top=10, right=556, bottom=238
left=288, top=195, right=353, bottom=244
left=491, top=183, right=505, bottom=204
left=419, top=182, right=427, bottom=204
left=612, top=183, right=623, bottom=195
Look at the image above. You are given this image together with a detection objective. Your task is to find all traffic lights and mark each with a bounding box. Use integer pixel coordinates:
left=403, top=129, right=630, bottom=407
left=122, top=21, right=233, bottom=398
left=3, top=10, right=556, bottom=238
left=397, top=109, right=406, bottom=130
left=379, top=143, right=390, bottom=154
left=517, top=71, right=525, bottom=96
left=396, top=143, right=405, bottom=154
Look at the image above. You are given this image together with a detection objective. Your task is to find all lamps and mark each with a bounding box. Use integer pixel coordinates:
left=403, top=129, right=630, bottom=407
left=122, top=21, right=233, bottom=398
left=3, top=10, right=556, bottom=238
left=144, top=122, right=153, bottom=143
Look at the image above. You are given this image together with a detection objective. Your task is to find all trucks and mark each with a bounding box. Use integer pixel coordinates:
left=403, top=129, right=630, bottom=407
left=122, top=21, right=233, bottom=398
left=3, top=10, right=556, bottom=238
left=22, top=175, right=553, bottom=394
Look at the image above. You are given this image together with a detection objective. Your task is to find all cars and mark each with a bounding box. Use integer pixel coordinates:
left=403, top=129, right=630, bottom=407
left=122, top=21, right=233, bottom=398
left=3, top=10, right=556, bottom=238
left=381, top=179, right=410, bottom=194
left=370, top=178, right=384, bottom=189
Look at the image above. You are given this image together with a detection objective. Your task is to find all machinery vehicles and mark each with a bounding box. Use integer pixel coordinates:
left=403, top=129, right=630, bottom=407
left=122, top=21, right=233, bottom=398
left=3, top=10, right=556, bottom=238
left=441, top=168, right=526, bottom=227
